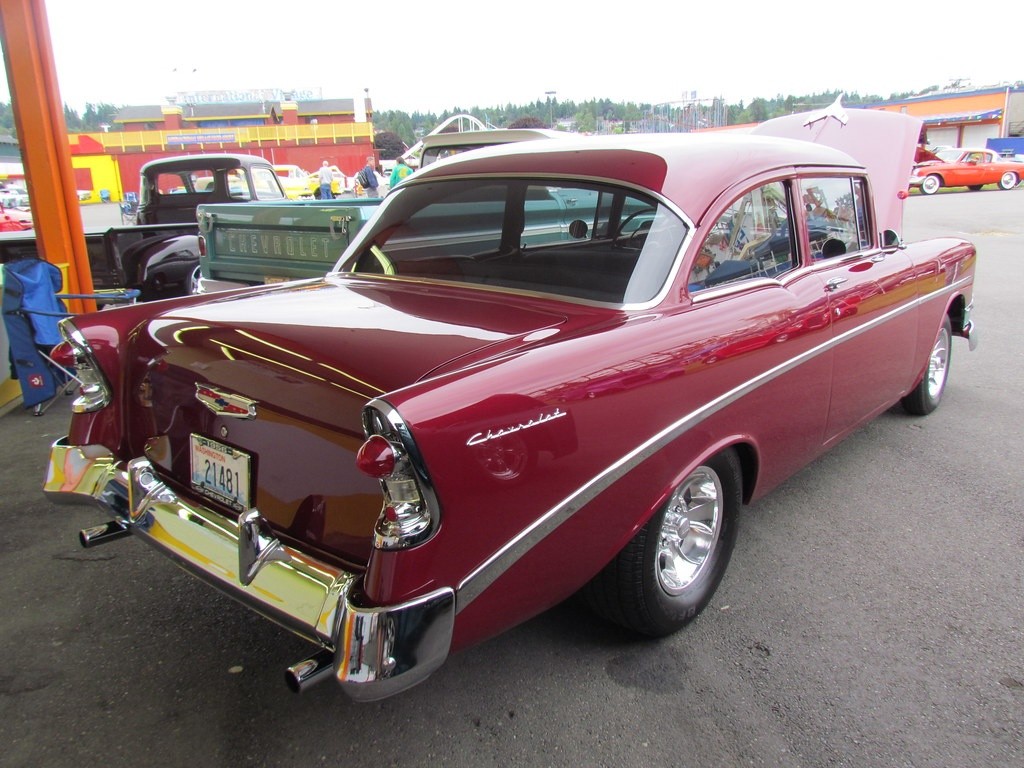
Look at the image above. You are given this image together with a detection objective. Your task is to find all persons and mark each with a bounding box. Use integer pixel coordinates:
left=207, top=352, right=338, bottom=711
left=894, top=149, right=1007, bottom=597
left=363, top=156, right=380, bottom=198
left=318, top=161, right=334, bottom=199
left=390, top=156, right=414, bottom=189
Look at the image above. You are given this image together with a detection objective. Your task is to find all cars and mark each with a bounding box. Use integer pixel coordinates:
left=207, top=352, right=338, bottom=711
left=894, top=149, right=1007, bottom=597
left=192, top=163, right=344, bottom=201
left=910, top=145, right=1024, bottom=196
left=0, top=181, right=35, bottom=230
left=42, top=95, right=981, bottom=704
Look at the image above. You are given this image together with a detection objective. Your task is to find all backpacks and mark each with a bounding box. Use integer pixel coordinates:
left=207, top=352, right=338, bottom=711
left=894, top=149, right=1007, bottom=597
left=357, top=165, right=370, bottom=189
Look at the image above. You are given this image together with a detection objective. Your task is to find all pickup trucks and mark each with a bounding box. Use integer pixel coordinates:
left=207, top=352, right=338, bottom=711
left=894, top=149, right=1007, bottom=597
left=0, top=152, right=295, bottom=301
left=195, top=129, right=593, bottom=297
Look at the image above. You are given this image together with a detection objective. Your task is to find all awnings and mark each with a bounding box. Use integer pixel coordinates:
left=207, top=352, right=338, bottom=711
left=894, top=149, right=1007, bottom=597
left=921, top=108, right=1003, bottom=124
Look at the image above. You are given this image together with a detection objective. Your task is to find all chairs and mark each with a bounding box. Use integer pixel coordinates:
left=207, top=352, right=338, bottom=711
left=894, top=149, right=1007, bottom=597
left=1, top=260, right=141, bottom=417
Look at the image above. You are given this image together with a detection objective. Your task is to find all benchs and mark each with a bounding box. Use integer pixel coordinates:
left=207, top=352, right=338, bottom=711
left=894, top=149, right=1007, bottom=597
left=517, top=249, right=642, bottom=294
